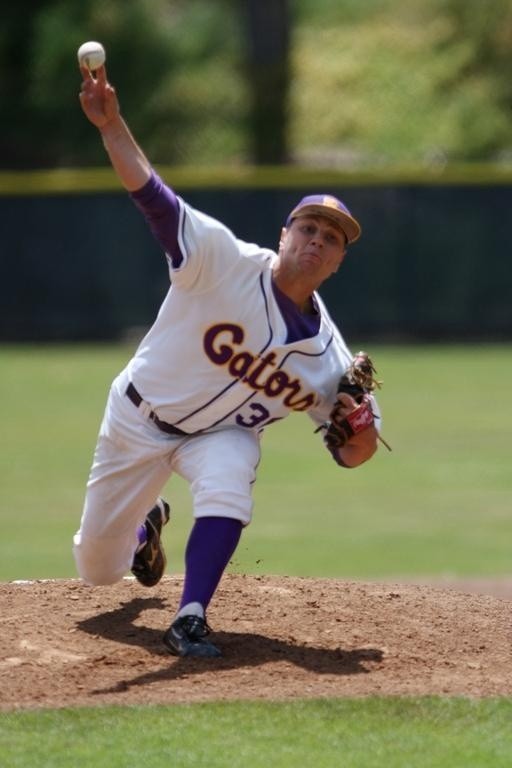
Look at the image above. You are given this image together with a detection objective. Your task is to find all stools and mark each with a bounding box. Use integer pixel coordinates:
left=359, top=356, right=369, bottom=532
left=125, top=382, right=188, bottom=436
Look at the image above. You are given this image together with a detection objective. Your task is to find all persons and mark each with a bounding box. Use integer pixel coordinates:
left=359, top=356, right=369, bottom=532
left=71, top=63, right=396, bottom=660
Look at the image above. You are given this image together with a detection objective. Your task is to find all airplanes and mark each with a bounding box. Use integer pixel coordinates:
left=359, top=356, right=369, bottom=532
left=314, top=351, right=390, bottom=449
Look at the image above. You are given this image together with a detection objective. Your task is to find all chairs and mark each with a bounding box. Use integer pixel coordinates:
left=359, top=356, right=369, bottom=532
left=161, top=614, right=224, bottom=662
left=130, top=498, right=170, bottom=587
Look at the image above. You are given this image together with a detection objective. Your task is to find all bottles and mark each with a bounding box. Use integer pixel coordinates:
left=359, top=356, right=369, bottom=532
left=286, top=194, right=362, bottom=245
